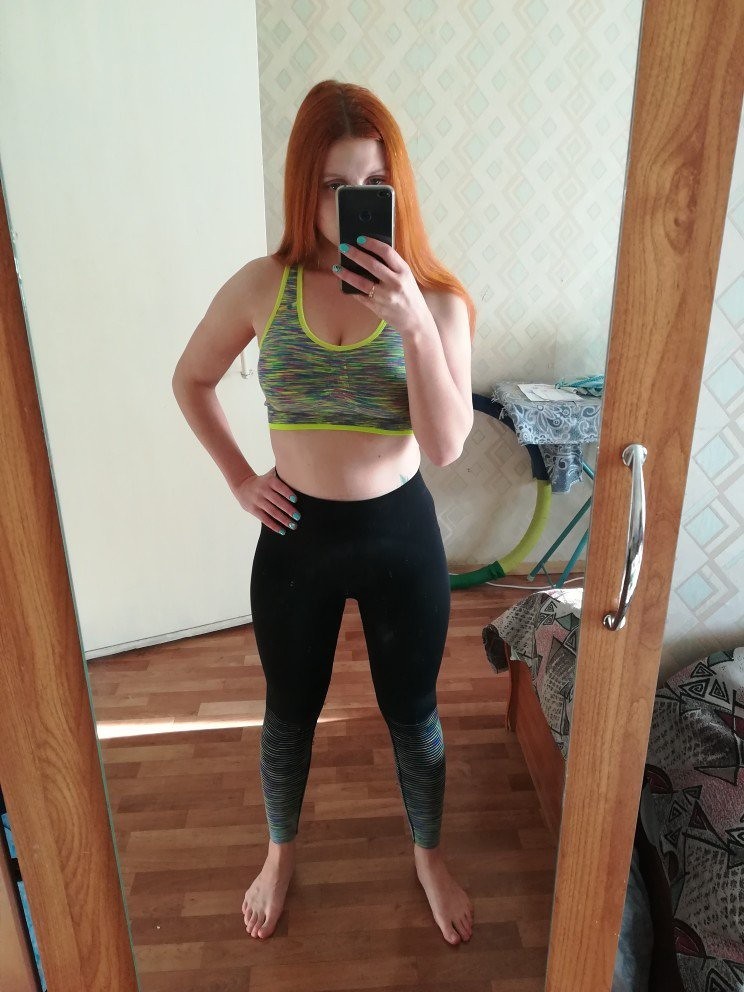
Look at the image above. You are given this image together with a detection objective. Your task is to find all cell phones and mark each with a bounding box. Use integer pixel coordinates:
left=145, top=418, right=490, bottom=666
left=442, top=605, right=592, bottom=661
left=337, top=186, right=394, bottom=294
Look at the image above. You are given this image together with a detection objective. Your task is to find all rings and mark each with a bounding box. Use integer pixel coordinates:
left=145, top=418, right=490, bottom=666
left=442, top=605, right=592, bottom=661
left=368, top=283, right=376, bottom=299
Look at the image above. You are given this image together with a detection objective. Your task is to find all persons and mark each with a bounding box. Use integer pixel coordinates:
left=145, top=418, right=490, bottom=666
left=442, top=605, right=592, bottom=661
left=171, top=77, right=479, bottom=946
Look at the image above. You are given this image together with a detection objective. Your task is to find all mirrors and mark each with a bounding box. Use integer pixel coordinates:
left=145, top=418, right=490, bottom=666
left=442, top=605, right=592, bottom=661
left=0, top=0, right=648, bottom=992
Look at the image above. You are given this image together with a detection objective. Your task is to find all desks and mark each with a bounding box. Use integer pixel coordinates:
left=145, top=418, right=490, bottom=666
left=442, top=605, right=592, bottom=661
left=490, top=380, right=605, bottom=589
